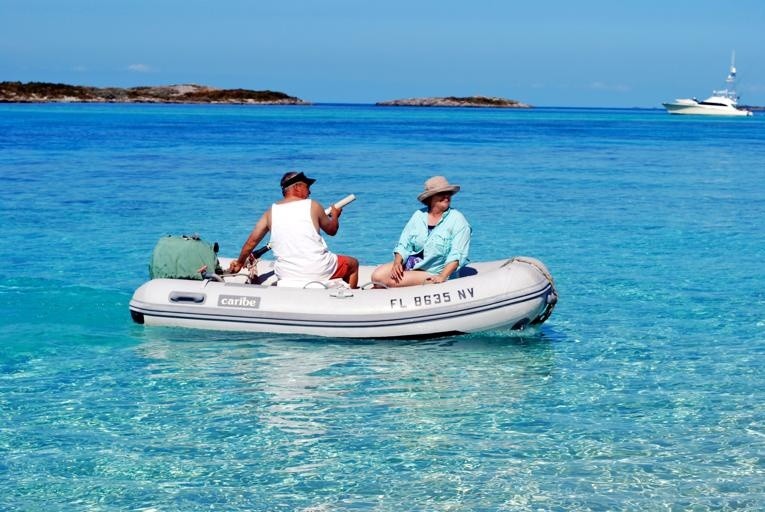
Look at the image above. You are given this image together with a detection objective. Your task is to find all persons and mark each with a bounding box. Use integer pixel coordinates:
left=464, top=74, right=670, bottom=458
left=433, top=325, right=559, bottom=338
left=229, top=171, right=358, bottom=289
left=370, top=175, right=478, bottom=289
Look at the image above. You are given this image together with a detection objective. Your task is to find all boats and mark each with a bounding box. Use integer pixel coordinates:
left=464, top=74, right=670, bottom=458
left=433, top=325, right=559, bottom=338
left=663, top=47, right=754, bottom=117
left=130, top=253, right=561, bottom=343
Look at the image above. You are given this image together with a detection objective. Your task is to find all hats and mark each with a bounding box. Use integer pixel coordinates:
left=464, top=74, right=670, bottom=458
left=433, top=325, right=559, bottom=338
left=417, top=176, right=460, bottom=201
left=281, top=171, right=316, bottom=190
